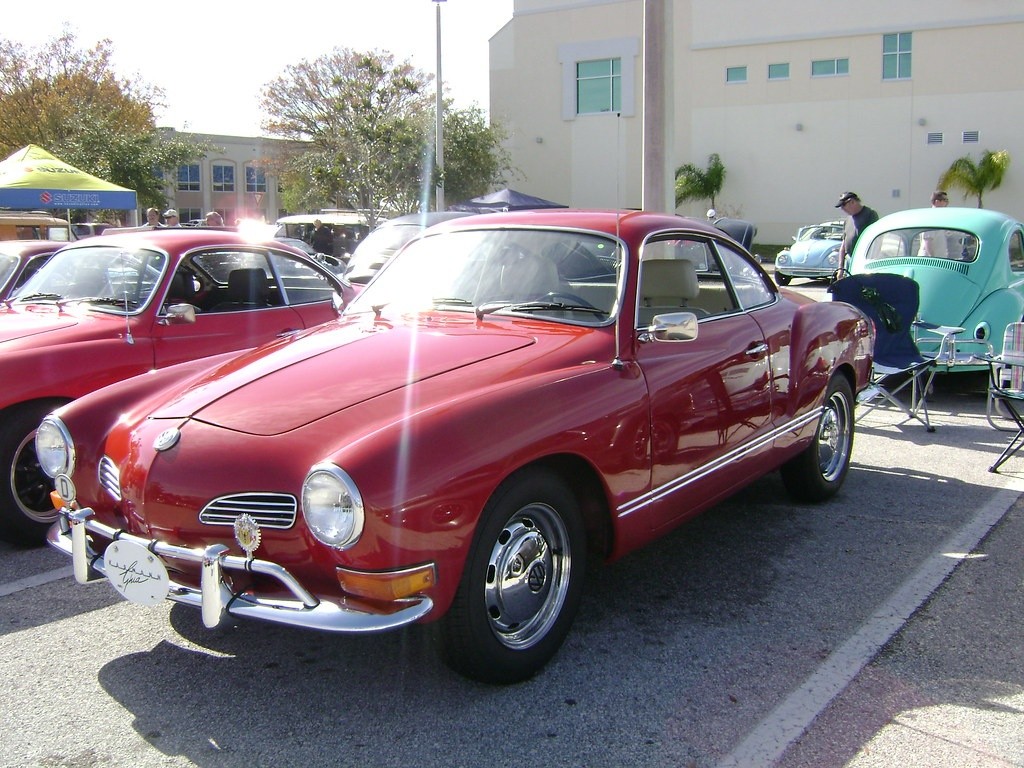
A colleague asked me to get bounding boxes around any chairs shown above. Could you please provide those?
[493,254,561,315]
[826,269,966,434]
[160,270,202,315]
[212,268,269,314]
[975,321,1024,474]
[636,259,711,330]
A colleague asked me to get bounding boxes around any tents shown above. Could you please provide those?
[451,188,569,212]
[0,144,140,241]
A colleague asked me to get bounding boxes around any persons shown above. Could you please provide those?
[706,209,718,220]
[835,192,883,281]
[310,219,334,256]
[163,209,182,227]
[141,208,165,227]
[918,191,950,259]
[206,212,226,226]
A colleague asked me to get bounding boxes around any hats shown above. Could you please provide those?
[162,209,179,217]
[834,191,857,207]
[707,209,716,217]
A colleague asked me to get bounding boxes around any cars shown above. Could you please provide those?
[1,229,367,551]
[253,237,347,277]
[35,209,880,688]
[0,239,200,302]
[774,221,845,287]
[846,208,1024,395]
[69,223,115,238]
[0,211,79,242]
[341,211,616,285]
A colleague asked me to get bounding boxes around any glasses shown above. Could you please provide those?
[843,198,850,207]
[165,215,176,219]
[938,198,948,202]
[709,215,715,219]
